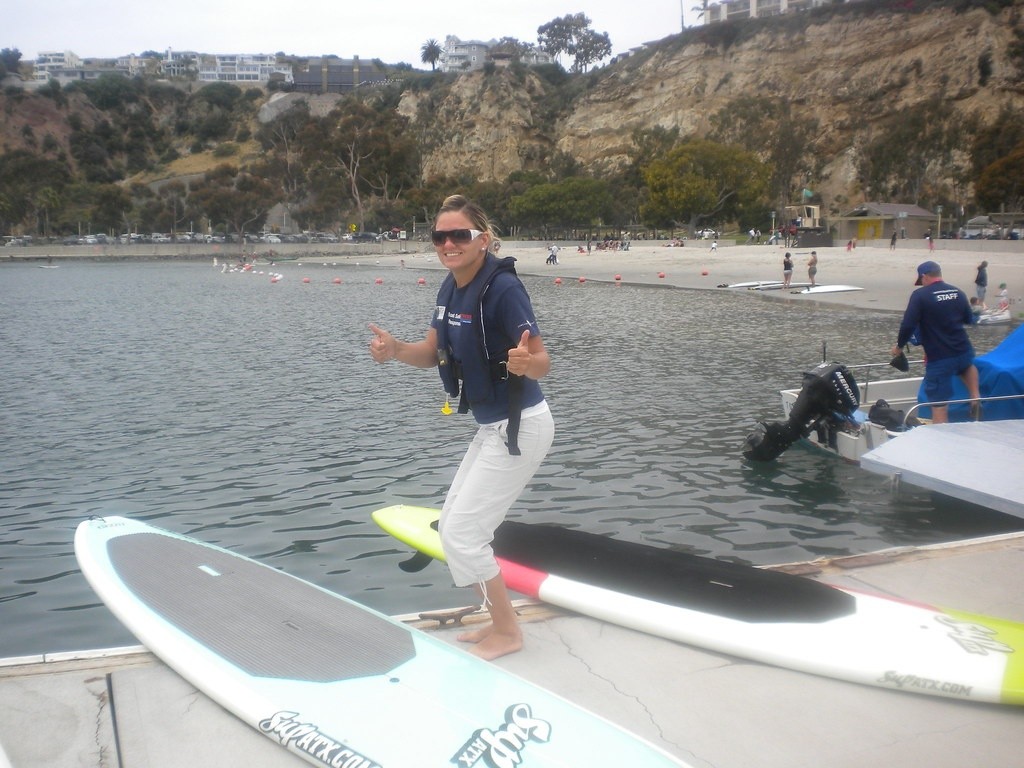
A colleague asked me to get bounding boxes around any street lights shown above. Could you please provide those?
[772,211,776,230]
[936,205,944,239]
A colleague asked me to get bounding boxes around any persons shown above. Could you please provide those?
[974,261,988,306]
[995,282,1008,310]
[781,252,794,290]
[890,232,897,250]
[552,243,558,264]
[927,224,1011,240]
[796,214,801,226]
[368,195,556,662]
[846,236,858,252]
[695,229,719,252]
[578,232,630,255]
[970,296,989,315]
[241,249,247,261]
[808,250,818,288]
[521,229,667,241]
[744,227,761,245]
[662,236,684,247]
[891,261,984,424]
[929,236,935,253]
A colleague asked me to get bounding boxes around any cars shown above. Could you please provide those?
[695,228,720,238]
[3,230,355,247]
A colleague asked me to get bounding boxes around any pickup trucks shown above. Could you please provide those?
[352,232,382,243]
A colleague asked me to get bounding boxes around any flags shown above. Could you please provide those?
[804,189,813,198]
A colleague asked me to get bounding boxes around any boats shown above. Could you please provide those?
[778,322,1024,462]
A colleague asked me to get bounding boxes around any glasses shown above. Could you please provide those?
[432,229,483,247]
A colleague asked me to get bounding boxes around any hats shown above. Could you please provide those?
[811,251,816,254]
[914,261,941,285]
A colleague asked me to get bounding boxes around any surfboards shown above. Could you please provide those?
[376,499,1022,709]
[751,283,821,290]
[73,513,697,768]
[799,285,864,294]
[728,281,785,288]
